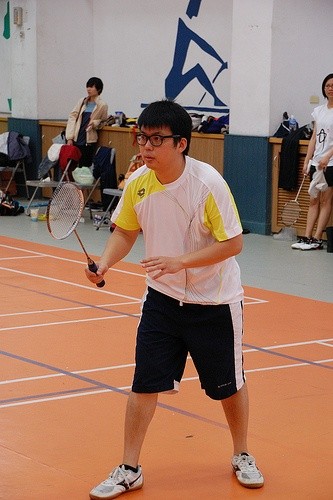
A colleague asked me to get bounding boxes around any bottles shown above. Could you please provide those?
[289,114,296,131]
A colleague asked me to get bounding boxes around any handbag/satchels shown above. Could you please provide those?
[118,161,146,191]
[0,191,24,216]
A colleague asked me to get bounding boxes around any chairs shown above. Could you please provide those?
[95,154,145,231]
[0,131,30,202]
[24,143,82,215]
[59,143,119,224]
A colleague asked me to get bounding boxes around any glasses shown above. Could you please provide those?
[135,132,180,146]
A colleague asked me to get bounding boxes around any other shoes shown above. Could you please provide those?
[85,199,103,211]
[93,214,110,226]
[274,228,297,241]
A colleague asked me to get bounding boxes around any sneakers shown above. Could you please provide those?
[231,452,264,488]
[89,465,143,500]
[291,236,324,250]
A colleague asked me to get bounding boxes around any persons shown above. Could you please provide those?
[66,77,108,169]
[291,74,333,251]
[84,101,264,500]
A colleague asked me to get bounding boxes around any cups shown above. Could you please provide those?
[31,208,39,222]
